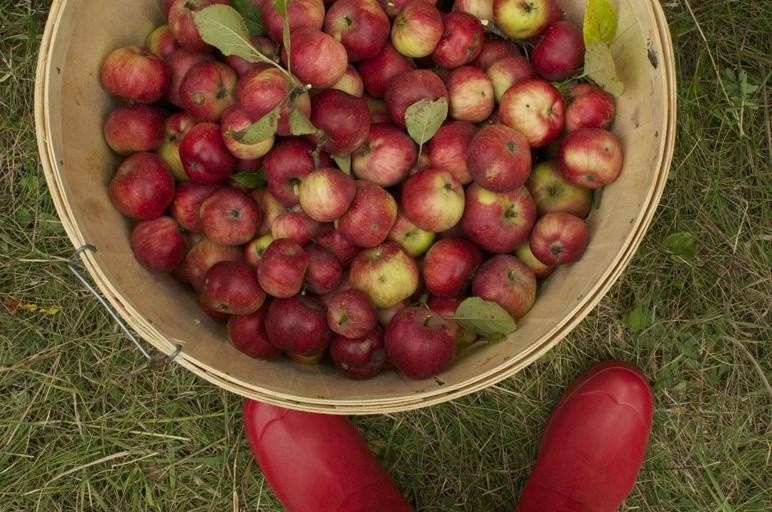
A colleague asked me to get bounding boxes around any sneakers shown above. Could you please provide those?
[513,360,657,511]
[238,396,421,512]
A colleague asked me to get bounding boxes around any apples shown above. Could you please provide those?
[101,0,623,379]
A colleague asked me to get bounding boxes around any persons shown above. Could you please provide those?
[242,357,655,511]
